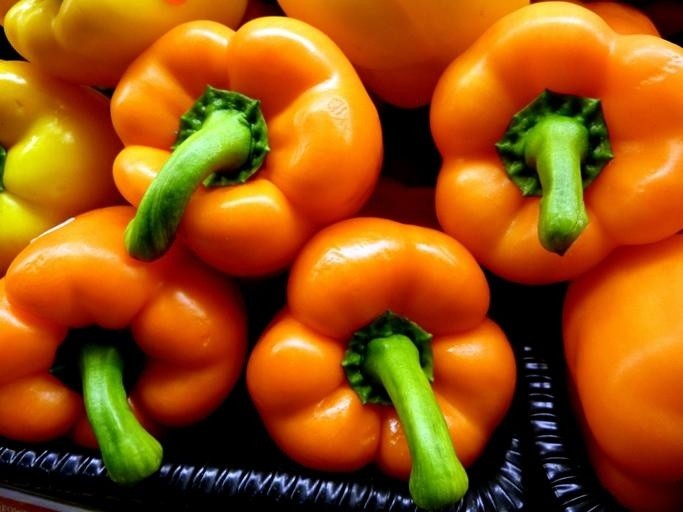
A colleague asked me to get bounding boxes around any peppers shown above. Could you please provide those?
[0,0,683,512]
